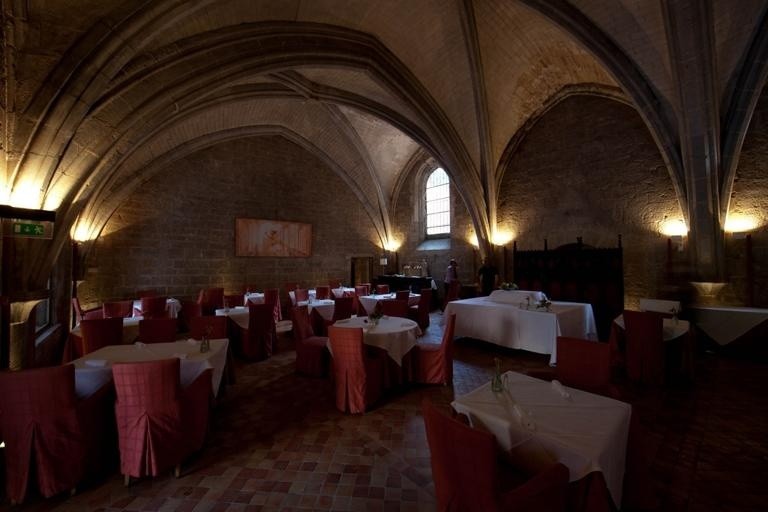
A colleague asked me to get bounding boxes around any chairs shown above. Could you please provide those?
[419,399,505,511]
[553,334,613,401]
[621,309,667,389]
[577,469,617,512]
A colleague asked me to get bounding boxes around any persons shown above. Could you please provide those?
[477,256,498,296]
[403,259,428,276]
[439,258,457,315]
[72,281,81,328]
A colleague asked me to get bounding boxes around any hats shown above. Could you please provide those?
[450,259,455,262]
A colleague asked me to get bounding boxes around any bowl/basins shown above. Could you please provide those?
[690,281,726,298]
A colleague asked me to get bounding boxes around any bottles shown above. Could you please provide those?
[492,372,503,390]
[504,373,509,390]
[520,295,552,312]
[199,336,210,352]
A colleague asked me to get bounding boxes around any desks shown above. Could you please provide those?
[607,312,689,397]
[445,369,634,511]
[443,289,599,368]
[690,305,768,364]
